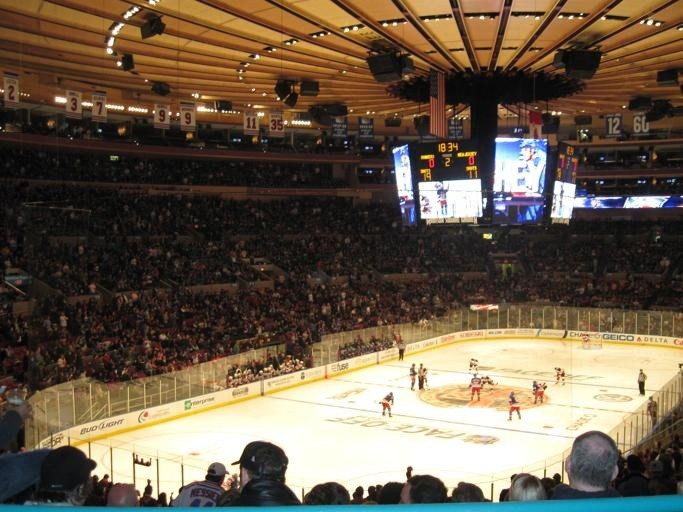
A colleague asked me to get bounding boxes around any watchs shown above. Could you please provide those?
[636,368,647,397]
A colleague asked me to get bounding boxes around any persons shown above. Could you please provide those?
[553,366,565,385]
[223,337,394,389]
[479,376,497,388]
[1,336,395,399]
[2,398,682,509]
[578,333,590,345]
[507,391,521,421]
[556,183,566,218]
[408,364,416,392]
[395,338,405,360]
[504,140,544,191]
[531,380,546,404]
[647,396,656,429]
[417,363,426,389]
[468,358,479,374]
[468,374,481,401]
[378,392,393,418]
[0,121,683,338]
[434,183,450,218]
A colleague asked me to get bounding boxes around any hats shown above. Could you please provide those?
[231,442,288,476]
[40,446,97,490]
[208,463,229,477]
